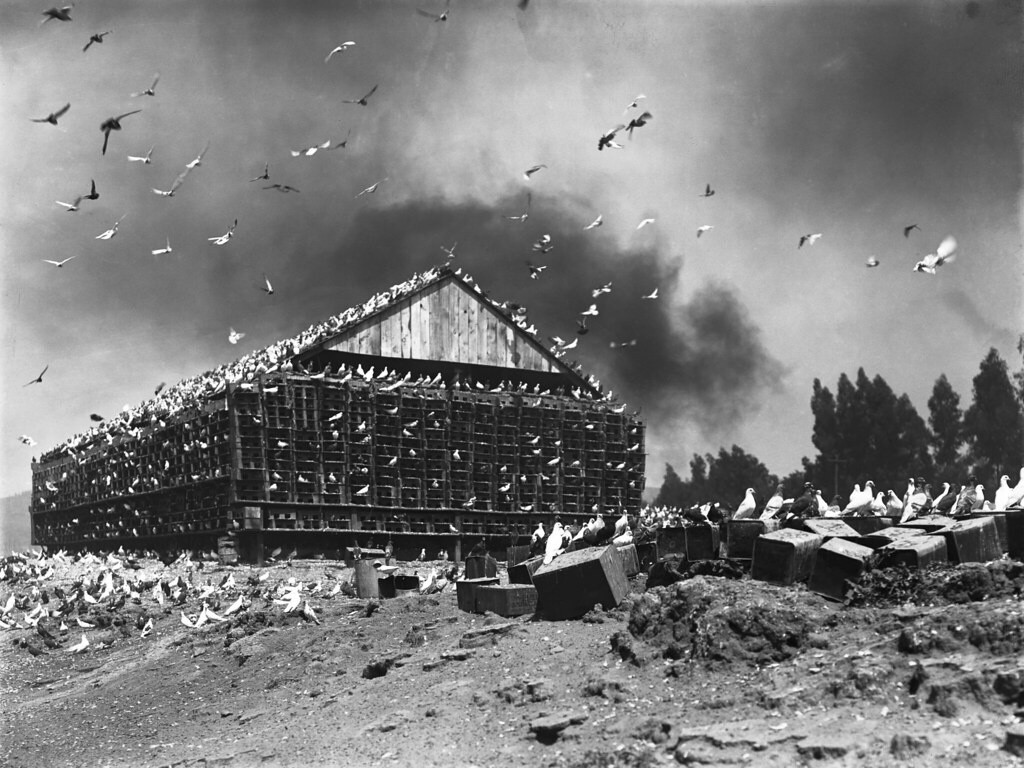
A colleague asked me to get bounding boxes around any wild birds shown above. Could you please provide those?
[28,102,71,126]
[129,73,159,97]
[41,162,388,295]
[341,84,378,106]
[0,261,1024,657]
[439,164,957,279]
[416,7,450,22]
[289,129,348,157]
[596,124,623,150]
[323,40,356,63]
[40,5,72,25]
[625,94,647,110]
[82,29,111,52]
[126,147,154,164]
[625,113,652,132]
[184,141,209,169]
[99,109,143,155]
